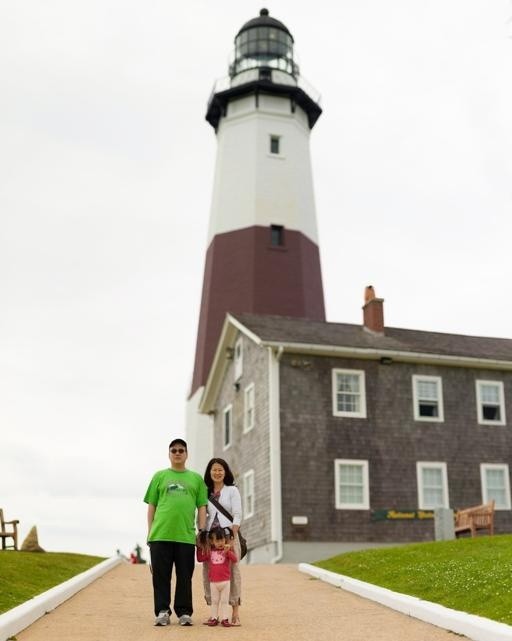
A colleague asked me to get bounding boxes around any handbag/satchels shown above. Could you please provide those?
[238,530,248,560]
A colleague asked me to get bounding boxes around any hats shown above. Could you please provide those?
[169,439,186,447]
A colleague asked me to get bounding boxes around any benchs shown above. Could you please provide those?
[453,499,496,538]
[0,508,19,550]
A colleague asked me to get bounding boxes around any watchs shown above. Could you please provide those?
[199,528,206,532]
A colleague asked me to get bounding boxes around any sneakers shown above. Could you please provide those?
[179,614,193,626]
[207,617,218,626]
[221,618,231,627]
[153,610,170,626]
[203,615,220,625]
[231,615,241,626]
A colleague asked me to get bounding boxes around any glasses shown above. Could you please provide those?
[170,448,184,454]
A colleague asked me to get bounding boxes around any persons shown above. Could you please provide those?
[197,527,238,627]
[196,458,242,626]
[144,439,212,626]
[134,543,142,559]
